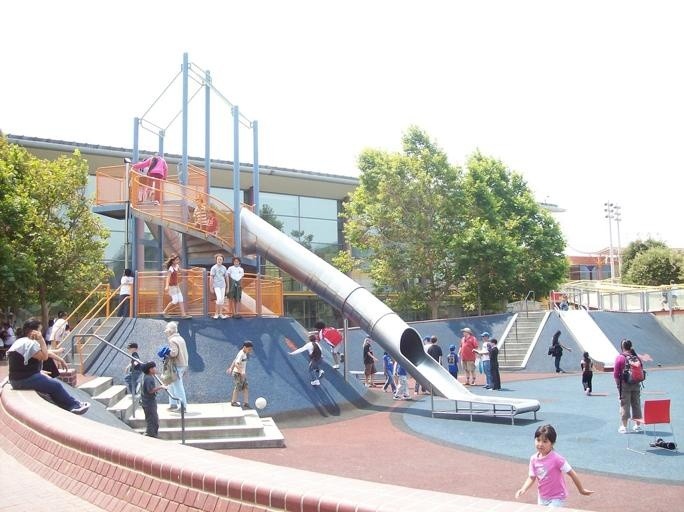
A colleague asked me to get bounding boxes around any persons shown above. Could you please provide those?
[613,338,642,434]
[193,198,219,239]
[288,334,325,386]
[209,254,229,319]
[661,280,680,311]
[0,312,91,414]
[551,330,572,373]
[226,340,253,410]
[315,320,345,369]
[559,291,570,311]
[124,321,188,437]
[227,256,245,318]
[580,352,594,396]
[118,267,134,317]
[130,152,169,206]
[160,251,192,320]
[515,424,594,508]
[363,327,502,401]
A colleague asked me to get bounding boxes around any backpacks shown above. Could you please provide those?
[621,354,647,385]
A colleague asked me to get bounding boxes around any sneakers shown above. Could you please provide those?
[617,425,629,435]
[339,353,345,363]
[585,387,590,394]
[632,424,643,433]
[70,401,91,415]
[332,364,340,369]
[162,311,172,319]
[318,369,324,378]
[213,313,220,319]
[167,405,178,412]
[231,401,241,407]
[242,402,252,410]
[403,395,412,401]
[181,314,193,320]
[392,395,402,401]
[174,407,187,413]
[310,379,321,386]
[219,313,227,320]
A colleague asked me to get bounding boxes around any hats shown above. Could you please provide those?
[480,332,490,336]
[460,328,473,334]
[448,344,456,351]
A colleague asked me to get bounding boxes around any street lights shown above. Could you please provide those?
[604,201,616,287]
[613,206,622,281]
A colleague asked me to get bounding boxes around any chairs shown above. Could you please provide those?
[626,399,680,457]
